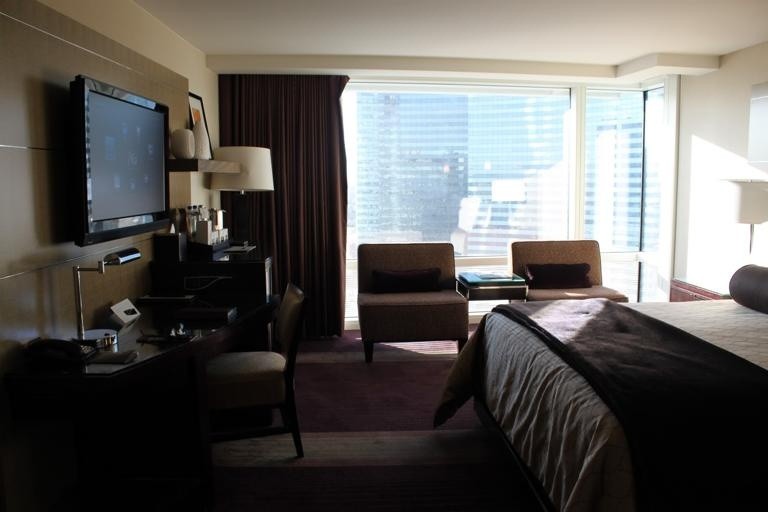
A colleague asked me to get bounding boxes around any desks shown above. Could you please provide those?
[77,295,281,511]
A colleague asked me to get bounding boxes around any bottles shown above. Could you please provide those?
[187,205,204,237]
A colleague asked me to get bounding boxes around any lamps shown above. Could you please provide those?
[71,247,143,349]
[728,178,768,255]
[208,145,275,253]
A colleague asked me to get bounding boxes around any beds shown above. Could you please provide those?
[471,300,767,510]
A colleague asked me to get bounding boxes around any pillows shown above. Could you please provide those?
[369,266,442,295]
[727,264,768,314]
[523,262,593,289]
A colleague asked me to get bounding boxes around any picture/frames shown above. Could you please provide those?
[188,92,214,159]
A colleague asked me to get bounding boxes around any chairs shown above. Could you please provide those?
[511,240,629,302]
[197,279,311,469]
[356,240,469,363]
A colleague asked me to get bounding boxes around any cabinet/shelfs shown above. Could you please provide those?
[147,253,273,345]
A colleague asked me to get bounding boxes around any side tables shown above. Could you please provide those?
[454,272,529,303]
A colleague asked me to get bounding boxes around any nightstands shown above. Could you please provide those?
[669,278,725,302]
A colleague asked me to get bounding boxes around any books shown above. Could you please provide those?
[472,269,512,279]
[458,271,525,287]
[223,245,257,253]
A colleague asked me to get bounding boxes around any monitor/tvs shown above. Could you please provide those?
[66,74,172,248]
